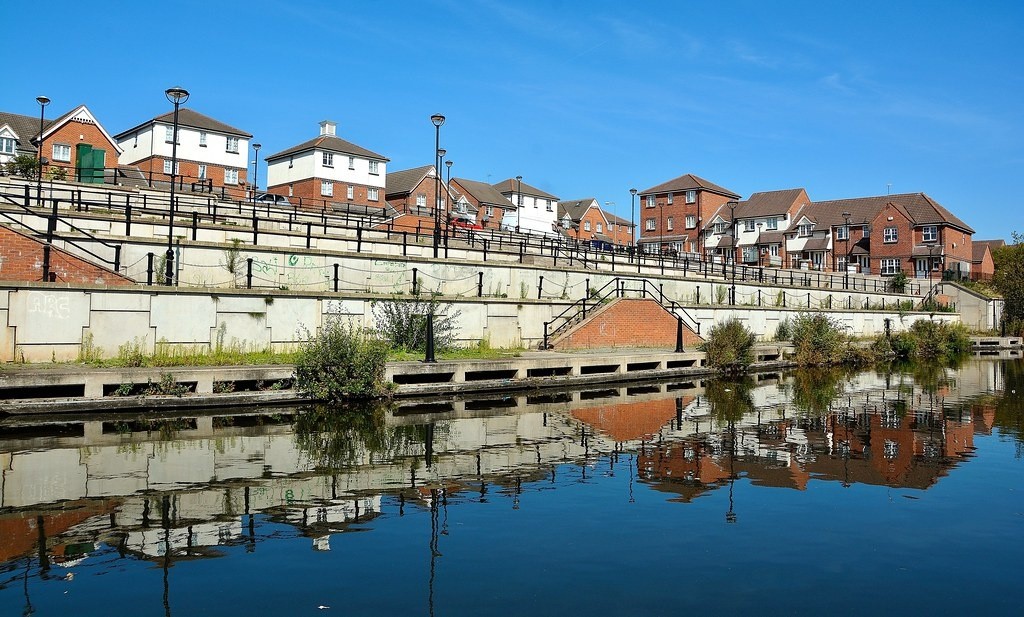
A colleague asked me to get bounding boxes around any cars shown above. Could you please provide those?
[244,192,293,207]
[447,216,485,229]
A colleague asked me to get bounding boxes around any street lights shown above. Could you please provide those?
[605,201,616,244]
[756,221,764,267]
[927,243,936,308]
[444,159,454,238]
[842,211,851,289]
[35,94,51,207]
[251,143,262,227]
[165,85,190,286]
[430,112,446,258]
[727,199,739,265]
[516,174,523,233]
[629,188,638,251]
[658,201,666,255]
[438,147,446,245]
